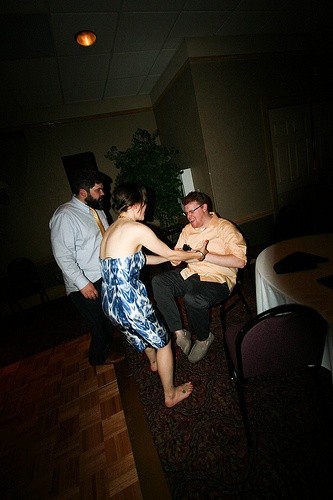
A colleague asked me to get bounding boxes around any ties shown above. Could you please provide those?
[90,206,105,237]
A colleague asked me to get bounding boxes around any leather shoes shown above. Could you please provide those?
[103,352,125,364]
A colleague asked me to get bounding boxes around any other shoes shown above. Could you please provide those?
[176,329,191,354]
[188,332,214,363]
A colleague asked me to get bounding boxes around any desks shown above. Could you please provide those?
[253,233,333,372]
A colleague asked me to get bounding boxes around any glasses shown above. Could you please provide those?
[182,203,204,216]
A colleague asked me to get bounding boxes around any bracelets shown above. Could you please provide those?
[196,250,205,261]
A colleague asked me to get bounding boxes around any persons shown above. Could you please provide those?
[100,182,209,407]
[49,170,125,364]
[152,192,247,364]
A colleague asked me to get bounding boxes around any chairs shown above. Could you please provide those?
[5,257,51,312]
[212,268,333,452]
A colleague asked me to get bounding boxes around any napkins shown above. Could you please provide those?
[273,249,329,275]
